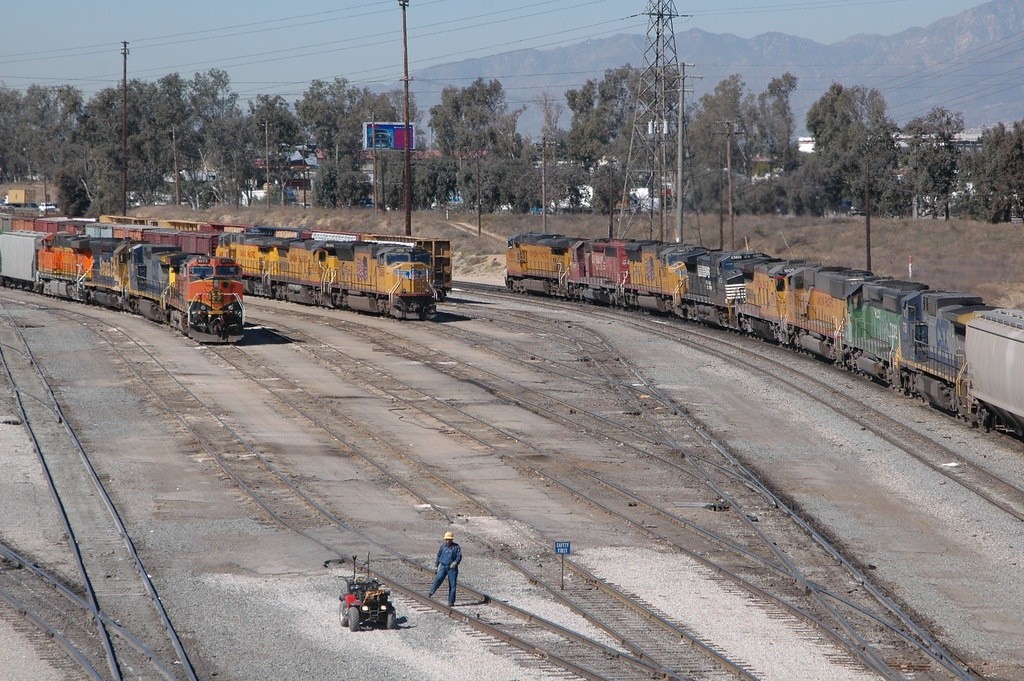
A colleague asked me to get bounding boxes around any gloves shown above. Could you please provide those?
[433,564,438,574]
[450,561,458,569]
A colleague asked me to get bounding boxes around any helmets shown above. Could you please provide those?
[444,531,454,539]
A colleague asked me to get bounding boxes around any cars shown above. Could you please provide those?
[39,202,56,214]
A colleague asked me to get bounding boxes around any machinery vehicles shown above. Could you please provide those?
[338,553,397,631]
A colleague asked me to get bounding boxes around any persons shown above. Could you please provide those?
[427,532,462,606]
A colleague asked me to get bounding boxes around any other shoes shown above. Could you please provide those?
[448,602,454,606]
[428,592,432,597]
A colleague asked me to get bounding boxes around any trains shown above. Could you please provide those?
[0,228,247,344]
[0,213,453,323]
[504,229,1024,440]
